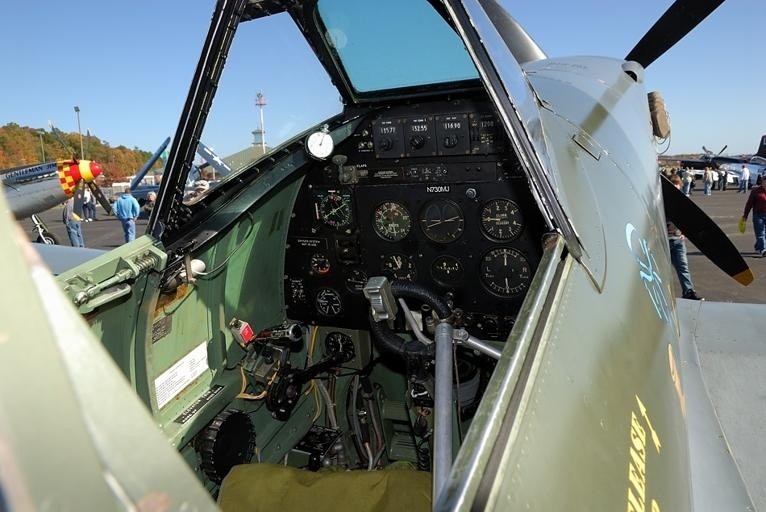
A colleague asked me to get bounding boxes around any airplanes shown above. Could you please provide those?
[2,158,104,221]
[0,0,764,512]
[680,144,766,186]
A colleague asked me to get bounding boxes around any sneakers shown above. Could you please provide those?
[681,290,702,300]
[761,249,766,257]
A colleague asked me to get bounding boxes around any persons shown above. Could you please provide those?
[182,180,210,201]
[112,188,141,243]
[62,187,100,223]
[200,166,208,180]
[663,175,705,301]
[142,191,157,217]
[63,197,85,248]
[742,176,766,256]
[659,165,730,197]
[737,165,750,193]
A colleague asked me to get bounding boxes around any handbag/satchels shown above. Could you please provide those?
[738,216,746,233]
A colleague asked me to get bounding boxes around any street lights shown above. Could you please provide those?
[72,105,85,158]
